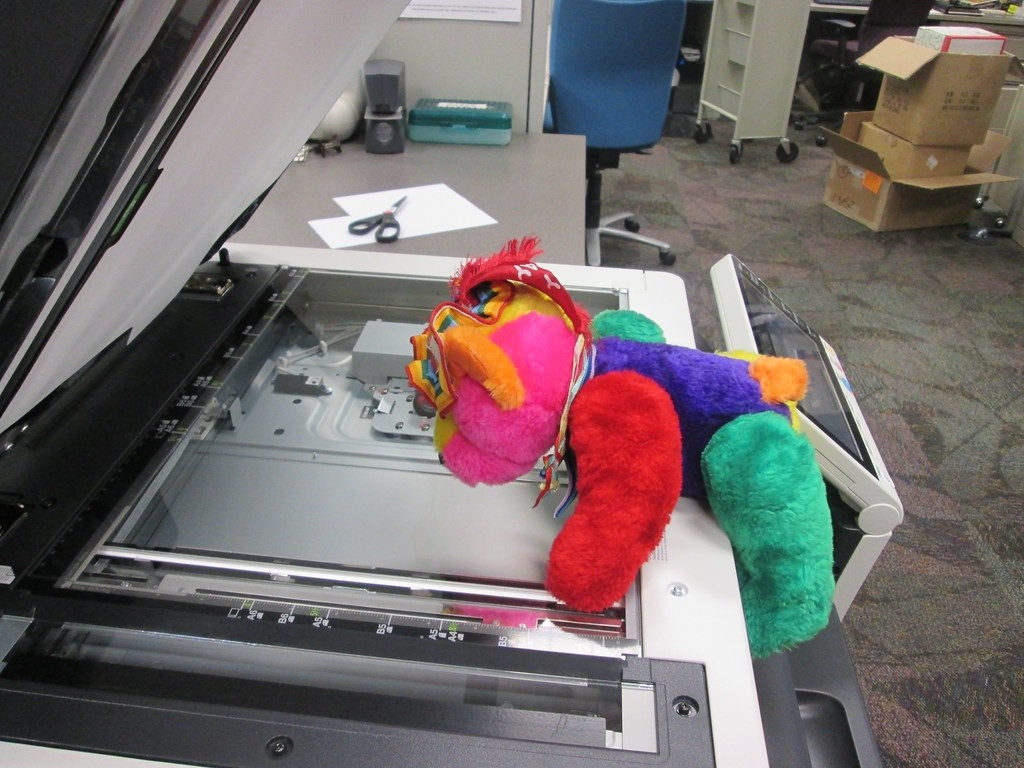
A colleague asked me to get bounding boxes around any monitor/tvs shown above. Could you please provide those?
[710,254,904,526]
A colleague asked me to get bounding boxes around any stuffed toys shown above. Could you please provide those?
[406,237,835,659]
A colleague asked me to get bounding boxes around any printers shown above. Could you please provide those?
[0,0,881,767]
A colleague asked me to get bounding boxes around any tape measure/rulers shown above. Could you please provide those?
[187,593,640,657]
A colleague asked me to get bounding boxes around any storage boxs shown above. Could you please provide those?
[855,36,1024,144]
[858,122,972,178]
[915,26,1007,54]
[819,112,1018,232]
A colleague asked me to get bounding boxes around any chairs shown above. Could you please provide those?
[542,0,686,267]
[794,0,936,147]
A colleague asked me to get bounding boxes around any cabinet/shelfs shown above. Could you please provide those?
[693,0,812,164]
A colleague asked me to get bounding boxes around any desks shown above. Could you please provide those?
[225,133,588,266]
[810,3,1024,26]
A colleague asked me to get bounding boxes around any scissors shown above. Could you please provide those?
[348,196,407,244]
